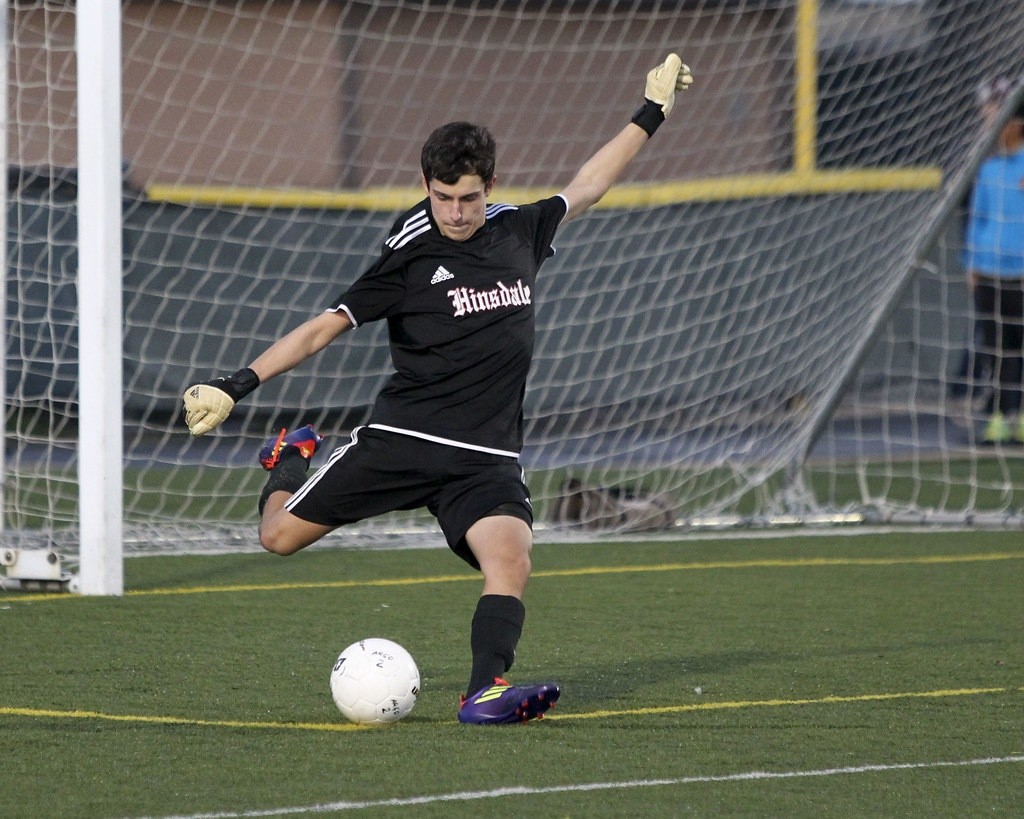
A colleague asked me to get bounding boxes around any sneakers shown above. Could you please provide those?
[257,424,324,470]
[457,677,561,726]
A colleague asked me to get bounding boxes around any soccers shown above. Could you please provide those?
[329,637,421,724]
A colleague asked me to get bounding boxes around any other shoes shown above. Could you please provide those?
[983,409,1024,444]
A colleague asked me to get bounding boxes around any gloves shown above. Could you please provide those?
[630,52,693,141]
[181,367,260,437]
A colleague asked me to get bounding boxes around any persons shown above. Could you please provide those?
[937,76,1024,446]
[183,52,694,726]
